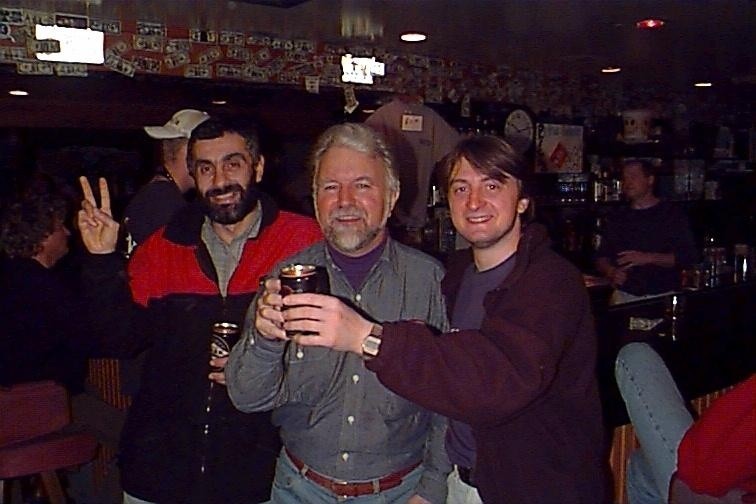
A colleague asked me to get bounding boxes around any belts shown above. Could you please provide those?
[284,447,422,497]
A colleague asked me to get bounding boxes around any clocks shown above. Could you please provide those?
[504,102,534,158]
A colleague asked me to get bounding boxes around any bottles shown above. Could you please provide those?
[589,215,607,258]
[587,154,625,202]
[732,243,750,284]
[454,113,497,136]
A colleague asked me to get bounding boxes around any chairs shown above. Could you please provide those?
[666,470,755,504]
[0,372,99,503]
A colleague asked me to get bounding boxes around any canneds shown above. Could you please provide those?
[280,263,320,340]
[208,321,241,373]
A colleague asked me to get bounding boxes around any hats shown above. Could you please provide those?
[144,110,209,139]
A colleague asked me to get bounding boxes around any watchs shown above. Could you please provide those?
[359,319,385,360]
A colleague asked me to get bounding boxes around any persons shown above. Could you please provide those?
[75,114,329,502]
[222,121,452,503]
[279,132,615,501]
[589,159,701,358]
[118,107,214,254]
[0,184,78,394]
